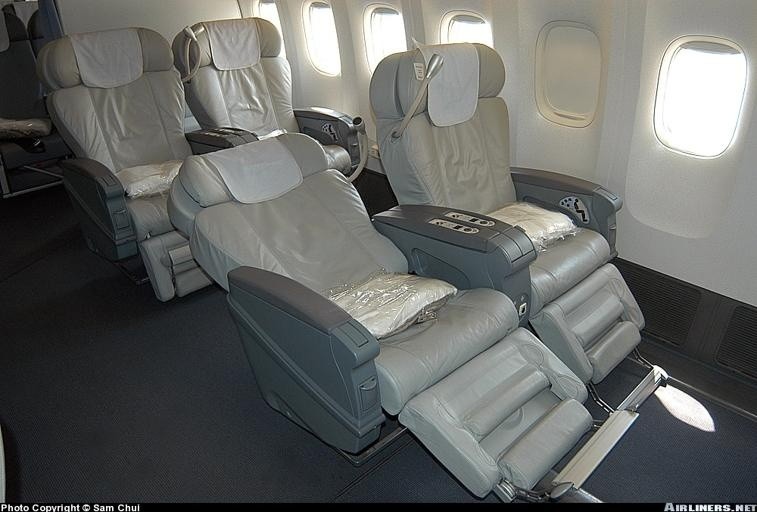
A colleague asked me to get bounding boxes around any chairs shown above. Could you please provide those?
[0,2,81,199]
[167,132,639,505]
[35,26,246,303]
[369,42,671,416]
[172,17,369,183]
